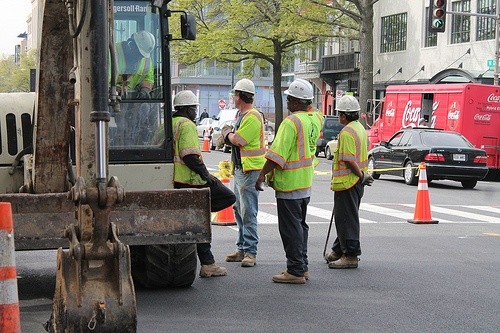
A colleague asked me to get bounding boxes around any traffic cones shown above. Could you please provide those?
[211,178,238,226]
[407,161,439,224]
[201,129,210,152]
[0,202,21,333]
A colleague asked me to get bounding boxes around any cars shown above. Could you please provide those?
[324,138,339,160]
[196,110,276,152]
[366,127,489,189]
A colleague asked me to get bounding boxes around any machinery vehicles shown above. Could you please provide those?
[0,0,211,333]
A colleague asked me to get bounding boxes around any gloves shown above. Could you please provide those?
[221,123,233,140]
[360,171,374,186]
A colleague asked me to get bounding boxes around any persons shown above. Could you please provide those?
[325,95,374,269]
[418,118,428,128]
[200,108,209,120]
[107,30,158,144]
[152,90,236,278]
[221,78,267,267]
[255,79,324,283]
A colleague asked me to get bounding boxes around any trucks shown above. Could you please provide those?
[360,82,500,181]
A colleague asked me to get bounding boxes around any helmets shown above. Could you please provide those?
[232,78,255,95]
[283,78,314,99]
[133,30,155,59]
[172,90,201,106]
[334,95,361,112]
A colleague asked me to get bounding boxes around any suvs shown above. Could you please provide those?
[314,114,346,157]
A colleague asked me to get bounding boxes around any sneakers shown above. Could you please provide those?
[241,253,256,267]
[225,250,245,262]
[199,263,227,277]
[325,251,341,263]
[328,254,360,268]
[272,271,309,284]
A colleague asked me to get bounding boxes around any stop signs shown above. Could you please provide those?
[218,99,226,109]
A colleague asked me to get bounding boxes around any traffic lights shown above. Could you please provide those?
[428,0,447,32]
